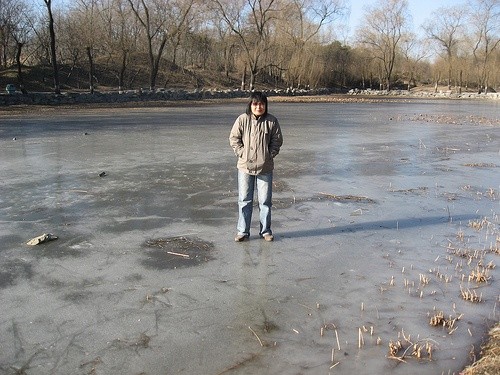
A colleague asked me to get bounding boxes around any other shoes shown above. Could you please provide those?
[262,232,273,242]
[235,235,249,242]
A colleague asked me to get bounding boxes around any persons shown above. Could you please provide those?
[229,92,283,242]
